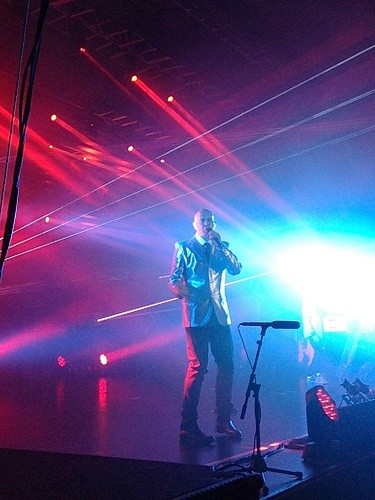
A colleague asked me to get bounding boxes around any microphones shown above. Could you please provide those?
[243,321,300,329]
[205,227,223,249]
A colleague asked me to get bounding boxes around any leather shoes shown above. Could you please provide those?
[216,415,243,437]
[180,416,216,443]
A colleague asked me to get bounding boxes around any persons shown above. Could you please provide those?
[167,208,244,443]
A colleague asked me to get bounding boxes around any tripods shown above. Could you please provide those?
[223,329,304,492]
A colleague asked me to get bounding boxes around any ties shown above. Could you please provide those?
[204,243,211,265]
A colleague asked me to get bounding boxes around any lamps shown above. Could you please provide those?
[340,377,374,409]
[302,386,348,461]
